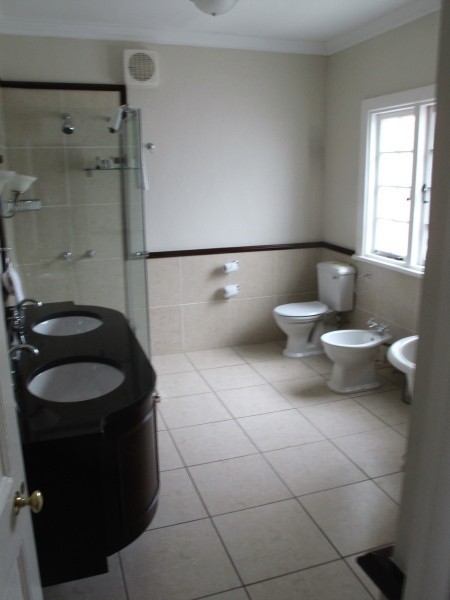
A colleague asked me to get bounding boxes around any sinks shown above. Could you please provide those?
[27,359,124,402]
[386,336,419,373]
[321,329,382,363]
[31,314,103,336]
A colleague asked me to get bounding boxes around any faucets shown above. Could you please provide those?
[367,318,384,335]
[7,345,38,360]
[9,299,42,339]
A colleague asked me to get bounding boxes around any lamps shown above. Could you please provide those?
[0,158,42,220]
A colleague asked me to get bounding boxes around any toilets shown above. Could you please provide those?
[272,261,356,358]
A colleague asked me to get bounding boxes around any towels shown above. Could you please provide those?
[2,268,23,302]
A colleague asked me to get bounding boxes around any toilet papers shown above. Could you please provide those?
[222,284,240,298]
[222,261,240,274]
